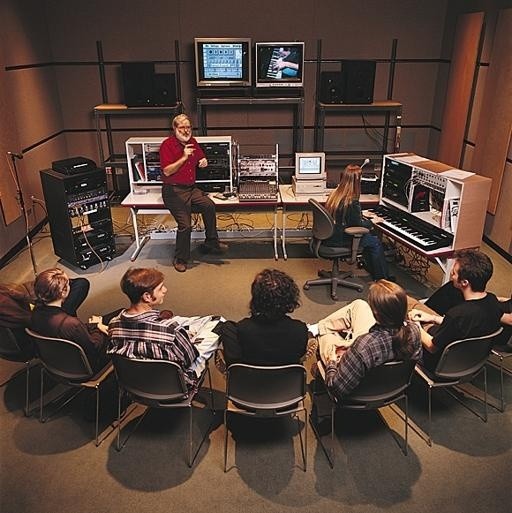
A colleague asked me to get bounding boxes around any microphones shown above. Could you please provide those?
[359,158,369,170]
[7,151,24,160]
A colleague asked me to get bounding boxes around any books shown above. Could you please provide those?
[162,314,227,354]
[131,155,146,182]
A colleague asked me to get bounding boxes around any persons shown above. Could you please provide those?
[272,48,300,77]
[322,163,397,281]
[31,268,126,383]
[222,269,308,414]
[107,268,213,403]
[0,278,90,361]
[159,113,229,272]
[306,278,421,406]
[407,249,504,384]
[424,280,512,346]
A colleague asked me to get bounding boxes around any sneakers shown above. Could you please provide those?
[299,338,318,365]
[213,350,226,375]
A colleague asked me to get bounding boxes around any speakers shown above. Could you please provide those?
[155,74,176,106]
[340,60,377,102]
[118,62,153,106]
[321,72,339,104]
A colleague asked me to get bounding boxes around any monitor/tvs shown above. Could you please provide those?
[254,41,305,88]
[193,36,252,88]
[295,152,324,180]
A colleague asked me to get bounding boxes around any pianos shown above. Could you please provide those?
[362,204,454,251]
[267,48,284,78]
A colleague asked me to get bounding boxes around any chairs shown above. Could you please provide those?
[221,363,309,474]
[413,326,505,448]
[24,327,138,448]
[109,353,218,468]
[0,325,77,418]
[309,358,417,471]
[490,347,512,414]
[302,198,364,302]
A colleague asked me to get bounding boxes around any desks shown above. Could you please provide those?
[119,191,282,263]
[277,183,380,262]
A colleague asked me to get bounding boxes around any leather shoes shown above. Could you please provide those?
[205,242,228,249]
[173,257,187,271]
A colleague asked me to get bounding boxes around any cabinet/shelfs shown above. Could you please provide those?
[92,102,185,207]
[315,101,405,168]
[361,151,493,305]
[195,97,306,171]
